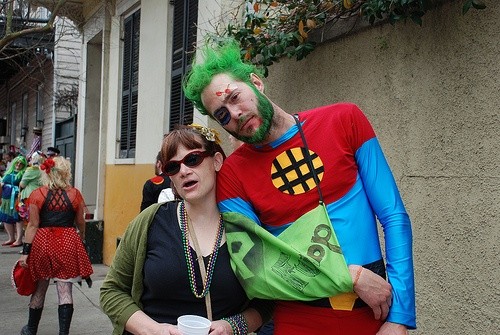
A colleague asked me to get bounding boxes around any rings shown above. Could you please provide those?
[21,261,24,264]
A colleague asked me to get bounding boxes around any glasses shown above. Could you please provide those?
[162,150,209,176]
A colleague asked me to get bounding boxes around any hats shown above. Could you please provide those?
[32,127,42,133]
[46,147,60,155]
[11,259,36,296]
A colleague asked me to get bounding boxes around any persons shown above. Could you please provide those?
[140,152,176,212]
[15,150,58,224]
[0,155,27,247]
[11,155,94,335]
[0,147,61,178]
[181,38,417,335]
[101,123,277,335]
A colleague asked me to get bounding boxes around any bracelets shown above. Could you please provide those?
[222,314,248,335]
[354,266,363,287]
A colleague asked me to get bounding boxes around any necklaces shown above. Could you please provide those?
[180,200,224,298]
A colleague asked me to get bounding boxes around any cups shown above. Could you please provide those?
[177,315,212,335]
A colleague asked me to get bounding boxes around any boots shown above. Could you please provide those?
[21,307,43,335]
[58,303,74,335]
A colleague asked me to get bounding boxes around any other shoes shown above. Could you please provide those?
[2,240,16,246]
[10,241,23,247]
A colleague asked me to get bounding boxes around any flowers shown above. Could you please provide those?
[39,158,56,174]
